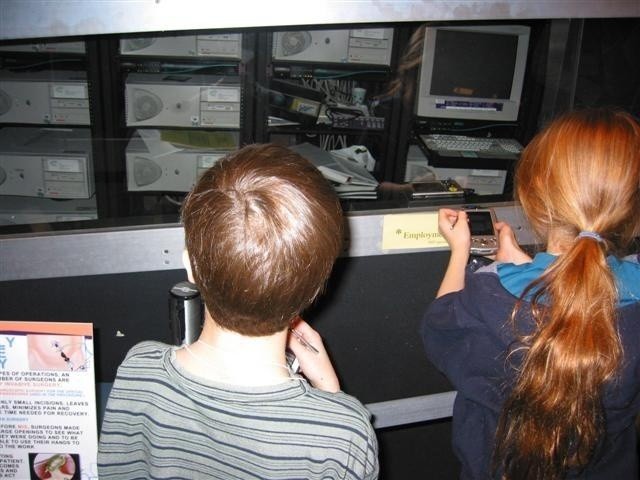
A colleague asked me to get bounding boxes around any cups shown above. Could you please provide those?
[169,283,200,346]
[351,87,366,104]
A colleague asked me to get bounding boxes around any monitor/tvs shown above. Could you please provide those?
[413,26,532,122]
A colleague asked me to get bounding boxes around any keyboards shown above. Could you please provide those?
[418,131,524,161]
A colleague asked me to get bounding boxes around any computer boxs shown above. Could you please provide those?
[118,34,242,60]
[0,68,91,126]
[403,144,508,198]
[270,28,395,68]
[0,127,96,201]
[122,72,239,131]
[124,128,239,193]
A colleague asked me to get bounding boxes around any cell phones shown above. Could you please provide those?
[462,206,500,257]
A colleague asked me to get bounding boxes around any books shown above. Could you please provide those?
[289,141,378,201]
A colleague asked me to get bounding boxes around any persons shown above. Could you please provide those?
[420,107,640,480]
[97,144,379,480]
[369,28,456,197]
